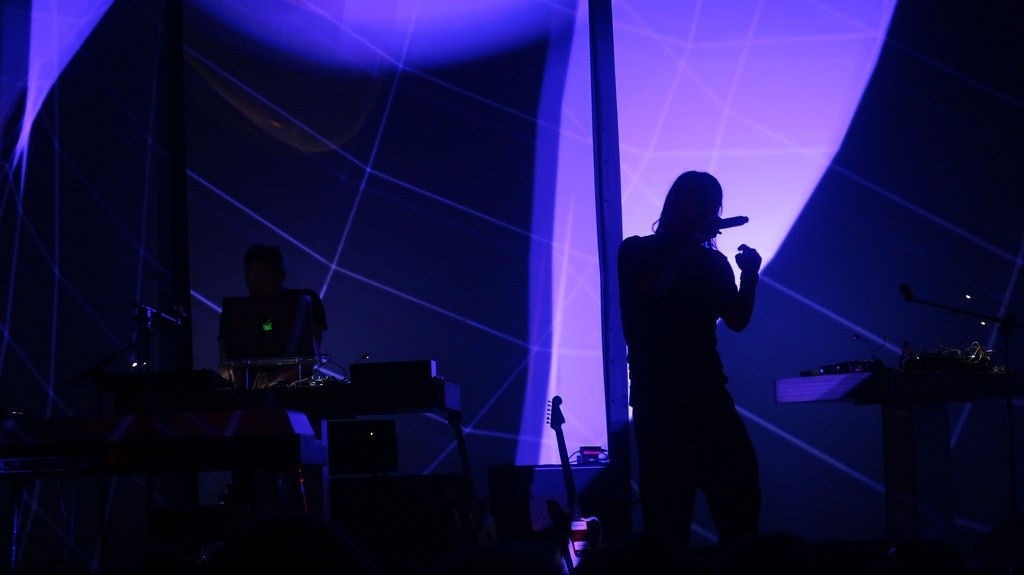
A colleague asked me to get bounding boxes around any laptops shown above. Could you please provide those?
[222,293,320,360]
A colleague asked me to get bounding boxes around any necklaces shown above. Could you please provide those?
[263,319,273,330]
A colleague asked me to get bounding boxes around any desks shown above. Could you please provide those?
[774,373,1023,544]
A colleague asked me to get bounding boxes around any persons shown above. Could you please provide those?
[218,245,326,390]
[617,171,761,547]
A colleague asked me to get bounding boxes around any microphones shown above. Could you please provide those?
[719,215,749,230]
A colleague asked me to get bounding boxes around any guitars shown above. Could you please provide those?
[544,393,600,574]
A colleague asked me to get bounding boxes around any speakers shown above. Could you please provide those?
[488,463,606,539]
[328,419,479,559]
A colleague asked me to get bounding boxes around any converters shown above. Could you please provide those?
[576,446,601,464]
